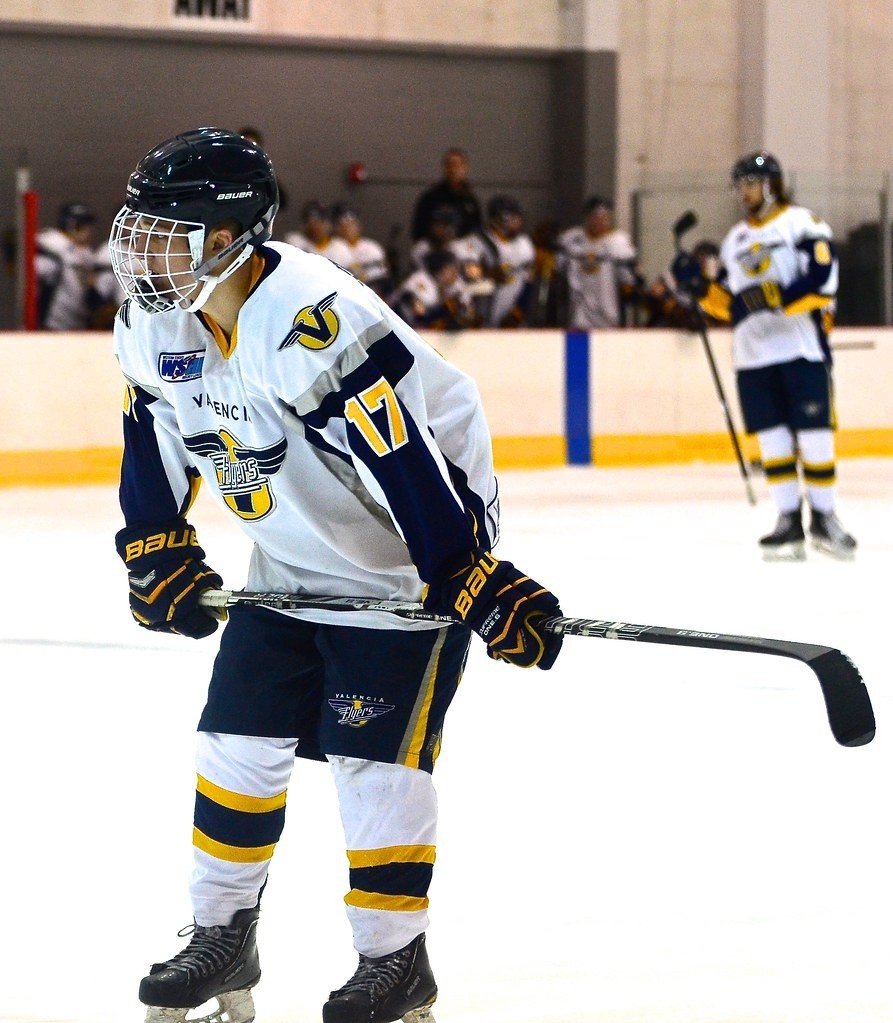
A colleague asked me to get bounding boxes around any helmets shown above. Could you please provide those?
[106,126,280,316]
[731,151,781,186]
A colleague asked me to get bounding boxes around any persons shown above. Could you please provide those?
[670,153,860,561]
[116,128,566,1023]
[1,145,732,332]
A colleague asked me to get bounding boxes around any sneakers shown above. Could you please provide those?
[757,497,806,562]
[809,508,858,561]
[322,932,438,1023]
[139,873,269,1023]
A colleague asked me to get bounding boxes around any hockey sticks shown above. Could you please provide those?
[191,583,878,746]
[669,206,760,509]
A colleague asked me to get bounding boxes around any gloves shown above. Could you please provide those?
[114,518,228,640]
[671,252,710,297]
[421,547,564,670]
